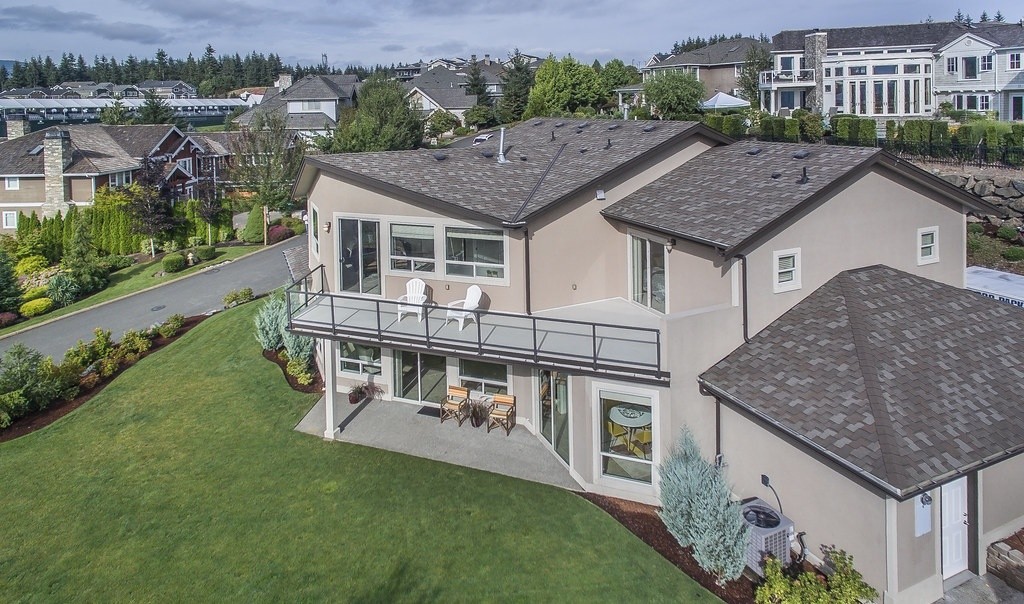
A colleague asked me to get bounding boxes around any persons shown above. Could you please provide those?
[302,212,308,232]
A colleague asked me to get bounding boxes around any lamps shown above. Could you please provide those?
[920,494,933,506]
[323,221,330,233]
[665,238,676,254]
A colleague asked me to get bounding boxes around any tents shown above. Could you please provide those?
[698,90,751,116]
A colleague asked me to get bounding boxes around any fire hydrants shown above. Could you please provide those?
[187,254,194,265]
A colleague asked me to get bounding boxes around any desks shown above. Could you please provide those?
[608,404,652,451]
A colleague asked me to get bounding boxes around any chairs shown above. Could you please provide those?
[607,419,627,447]
[440,385,469,427]
[357,346,381,373]
[630,430,652,459]
[445,285,482,332]
[397,277,427,323]
[486,394,515,436]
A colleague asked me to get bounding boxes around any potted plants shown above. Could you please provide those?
[349,383,385,404]
[460,399,491,429]
